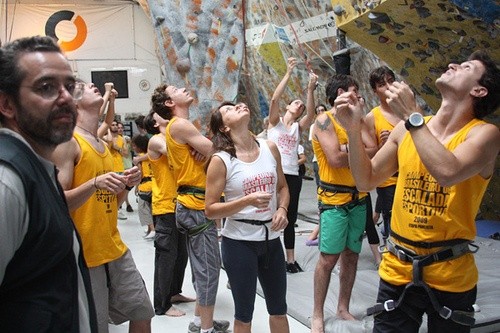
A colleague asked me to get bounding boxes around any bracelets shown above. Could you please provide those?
[125,185,134,191]
[280,206,288,212]
[95,178,99,189]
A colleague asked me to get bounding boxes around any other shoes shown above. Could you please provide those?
[127,205,133,212]
[117,209,127,219]
[145,230,155,239]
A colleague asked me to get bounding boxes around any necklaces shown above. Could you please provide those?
[76,125,100,142]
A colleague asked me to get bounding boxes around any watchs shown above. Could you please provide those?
[118,148,121,152]
[405,112,424,129]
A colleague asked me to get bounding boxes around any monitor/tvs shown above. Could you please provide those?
[90,71,129,99]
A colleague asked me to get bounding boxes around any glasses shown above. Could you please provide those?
[16,83,85,101]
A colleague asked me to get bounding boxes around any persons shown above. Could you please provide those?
[145,110,197,316]
[365,65,425,244]
[205,120,233,289]
[309,104,328,186]
[258,57,317,273]
[51,78,156,333]
[334,49,500,333]
[311,74,378,333]
[0,35,100,333]
[152,85,233,333]
[204,102,290,333]
[98,82,155,239]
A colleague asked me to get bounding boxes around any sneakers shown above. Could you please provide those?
[187,320,230,333]
[285,260,303,273]
